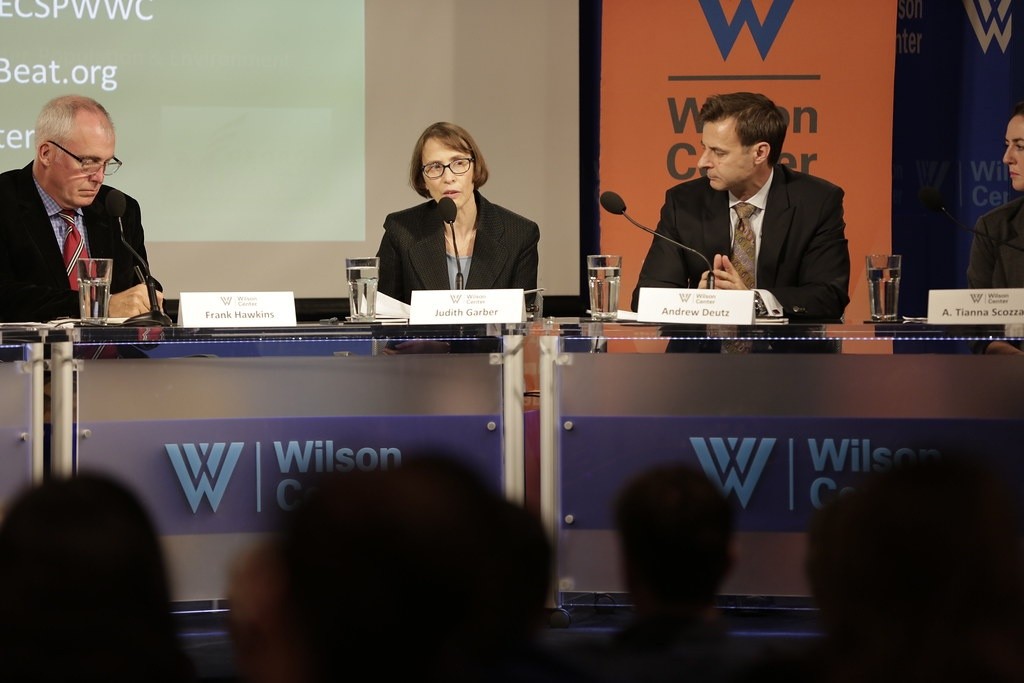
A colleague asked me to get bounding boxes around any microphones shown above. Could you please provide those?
[105,190,172,328]
[437,196,464,290]
[598,191,715,289]
[918,187,1024,251]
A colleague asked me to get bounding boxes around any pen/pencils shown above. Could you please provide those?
[134,266,146,285]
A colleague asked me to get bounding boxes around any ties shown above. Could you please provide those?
[705,324,737,338]
[135,325,163,341]
[720,202,756,352]
[57,209,120,359]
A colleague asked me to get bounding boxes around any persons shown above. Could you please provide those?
[967,101,1024,289]
[377,122,540,308]
[229,454,555,683]
[567,461,743,683]
[632,92,851,324]
[0,473,194,683]
[807,449,1024,683]
[0,95,164,323]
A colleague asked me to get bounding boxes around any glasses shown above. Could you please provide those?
[45,140,122,176]
[424,158,474,179]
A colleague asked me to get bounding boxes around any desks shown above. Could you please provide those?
[530,312,1024,627]
[46,317,528,627]
[0,319,46,520]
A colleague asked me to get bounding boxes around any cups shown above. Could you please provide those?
[865,254,902,321]
[76,257,113,325]
[345,257,379,321]
[587,254,621,320]
